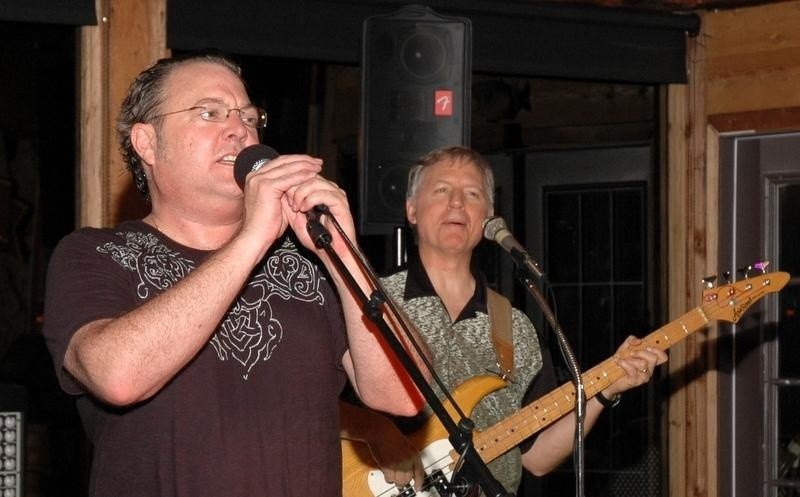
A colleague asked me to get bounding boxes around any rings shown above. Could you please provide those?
[640,369,647,374]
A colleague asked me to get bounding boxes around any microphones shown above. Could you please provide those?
[234,143,336,249]
[481,215,546,281]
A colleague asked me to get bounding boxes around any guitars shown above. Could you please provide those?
[341,261,790,497]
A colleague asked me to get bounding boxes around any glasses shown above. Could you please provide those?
[159,106,268,128]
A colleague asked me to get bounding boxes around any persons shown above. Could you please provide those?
[41,54,432,497]
[341,147,669,497]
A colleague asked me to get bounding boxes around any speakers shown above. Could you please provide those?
[358,6,473,234]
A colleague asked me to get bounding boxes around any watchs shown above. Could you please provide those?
[595,391,621,408]
